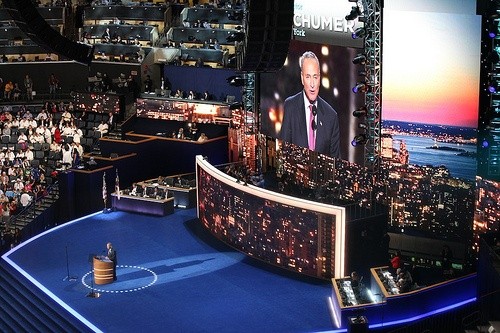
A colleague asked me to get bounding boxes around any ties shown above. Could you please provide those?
[309,105,316,151]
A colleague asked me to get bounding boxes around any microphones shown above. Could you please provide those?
[103,251,105,252]
[312,105,317,116]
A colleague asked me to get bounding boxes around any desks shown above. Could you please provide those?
[91,61,142,96]
[67,165,114,218]
[136,182,196,209]
[181,49,224,62]
[84,25,153,41]
[164,64,242,102]
[188,7,243,24]
[126,131,227,175]
[172,27,245,45]
[332,276,362,328]
[0,62,90,96]
[83,152,136,191]
[110,191,174,216]
[136,96,241,124]
[76,91,125,115]
[100,136,158,182]
[370,267,403,322]
[0,6,70,54]
[94,44,152,57]
[93,6,166,21]
[479,234,500,276]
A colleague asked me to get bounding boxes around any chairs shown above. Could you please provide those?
[0,106,110,189]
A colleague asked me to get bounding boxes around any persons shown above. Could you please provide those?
[351,272,358,286]
[280,51,341,160]
[158,175,184,188]
[0,101,115,232]
[173,128,208,142]
[390,251,408,293]
[107,242,117,281]
[0,0,244,103]
[129,182,172,199]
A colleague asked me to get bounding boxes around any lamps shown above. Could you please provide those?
[226,76,249,87]
[228,102,244,110]
[345,7,372,147]
[483,25,497,147]
